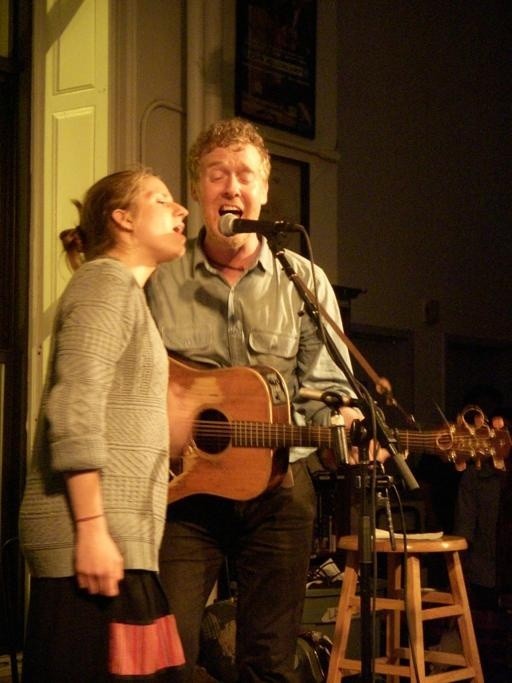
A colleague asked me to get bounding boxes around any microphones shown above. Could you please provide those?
[217,212,297,237]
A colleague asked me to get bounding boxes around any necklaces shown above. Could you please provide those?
[204,256,244,273]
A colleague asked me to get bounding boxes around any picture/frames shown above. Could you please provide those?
[232,1,317,257]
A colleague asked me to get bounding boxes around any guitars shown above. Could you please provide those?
[169,350,510,505]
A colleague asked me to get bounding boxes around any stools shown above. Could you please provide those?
[326,533,483,683]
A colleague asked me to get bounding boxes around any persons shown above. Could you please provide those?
[449,454,512,642]
[144,118,390,682]
[15,170,192,683]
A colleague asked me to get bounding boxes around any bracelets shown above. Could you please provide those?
[75,511,105,526]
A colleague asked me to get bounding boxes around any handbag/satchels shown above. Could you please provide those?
[306,558,360,587]
